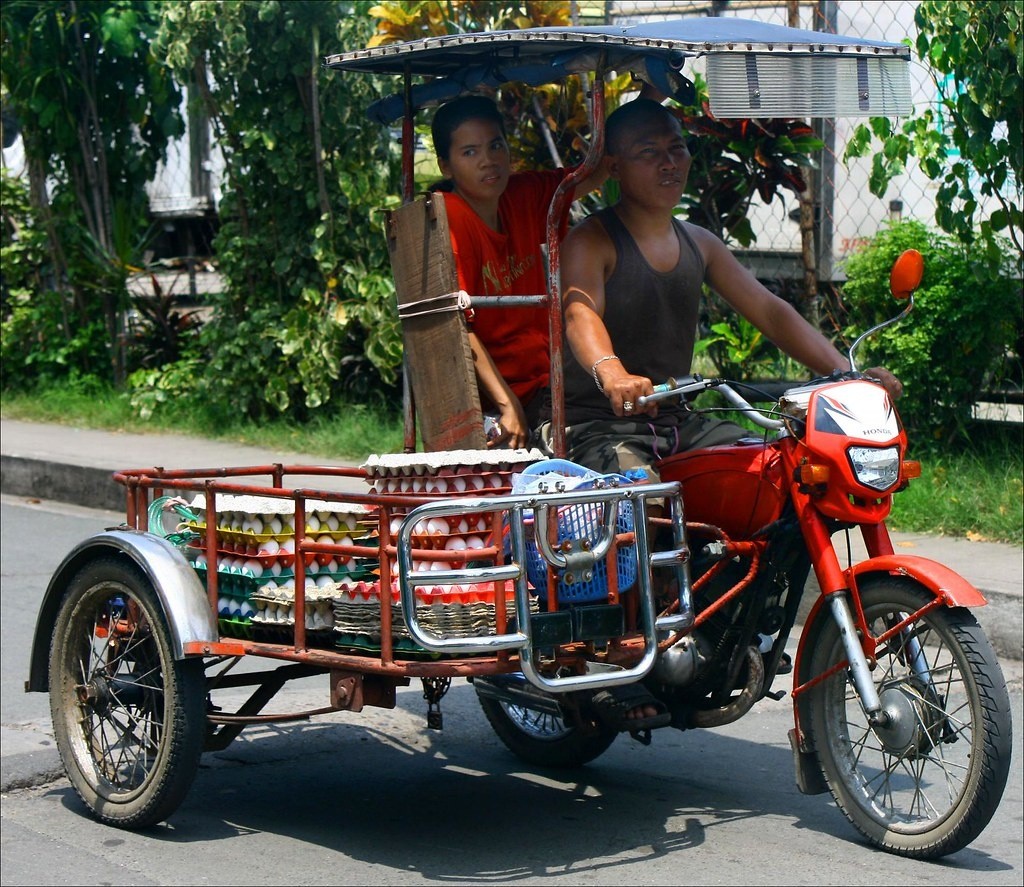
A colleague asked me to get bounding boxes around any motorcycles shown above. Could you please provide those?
[22,13,1015,859]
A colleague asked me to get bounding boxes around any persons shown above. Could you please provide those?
[431,84,675,451]
[560,97,902,730]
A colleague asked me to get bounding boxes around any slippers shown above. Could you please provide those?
[579,684,672,731]
[762,651,793,673]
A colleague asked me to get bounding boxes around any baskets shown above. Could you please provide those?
[508,459,637,602]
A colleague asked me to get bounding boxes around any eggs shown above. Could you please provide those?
[195,473,525,625]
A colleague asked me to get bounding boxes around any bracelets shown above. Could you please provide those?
[592,355,620,392]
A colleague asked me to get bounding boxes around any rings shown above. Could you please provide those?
[624,401,636,412]
